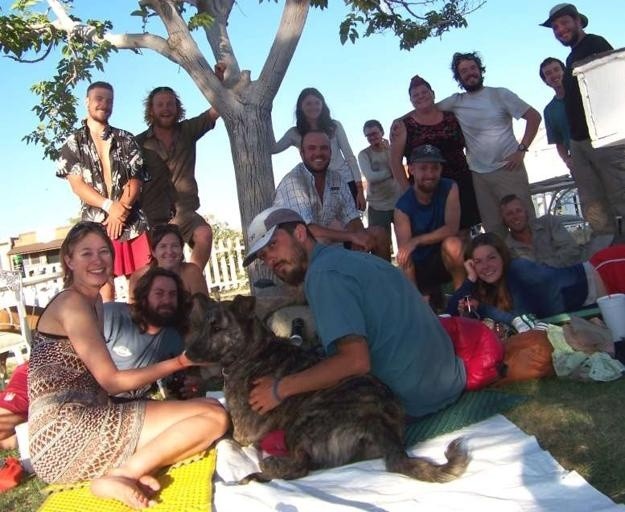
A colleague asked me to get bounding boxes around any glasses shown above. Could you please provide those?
[151,223,178,232]
[464,294,472,312]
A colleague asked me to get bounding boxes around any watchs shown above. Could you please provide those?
[518,144,526,151]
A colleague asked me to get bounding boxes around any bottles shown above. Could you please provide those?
[463,295,475,319]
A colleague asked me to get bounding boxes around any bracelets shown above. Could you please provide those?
[274,378,283,401]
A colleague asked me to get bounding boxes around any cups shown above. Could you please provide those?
[596,293,625,344]
[14,421,30,460]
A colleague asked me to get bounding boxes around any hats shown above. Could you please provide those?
[242,207,305,266]
[410,144,446,163]
[539,3,588,30]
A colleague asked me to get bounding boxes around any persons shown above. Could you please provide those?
[242,205,466,420]
[25,220,231,507]
[136,61,227,271]
[129,223,209,311]
[358,120,405,261]
[393,144,473,307]
[539,57,573,173]
[274,129,377,254]
[0,267,186,452]
[390,52,541,240]
[541,3,625,239]
[446,231,625,323]
[272,88,367,212]
[389,75,482,233]
[498,194,582,269]
[56,81,152,303]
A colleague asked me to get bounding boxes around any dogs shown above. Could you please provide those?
[183,293,475,485]
